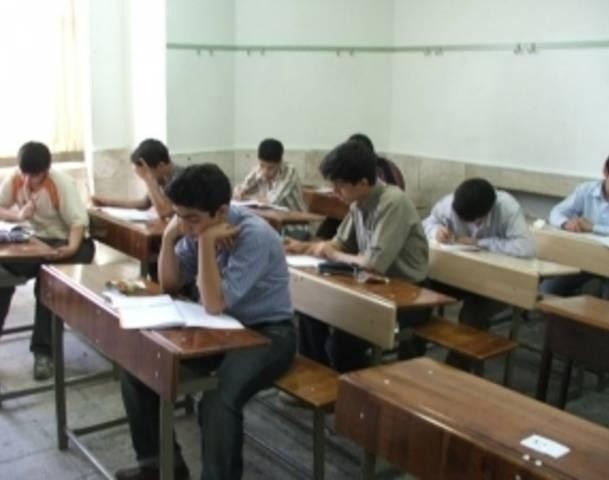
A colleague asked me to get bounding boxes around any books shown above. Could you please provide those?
[235,198,283,210]
[115,294,245,331]
[103,203,160,221]
[1,221,28,240]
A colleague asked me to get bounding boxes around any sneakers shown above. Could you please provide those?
[112,456,191,480]
[33,354,54,381]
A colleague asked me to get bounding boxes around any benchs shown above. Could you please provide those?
[276,353,345,479]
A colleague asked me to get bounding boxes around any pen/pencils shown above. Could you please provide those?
[282,229,286,244]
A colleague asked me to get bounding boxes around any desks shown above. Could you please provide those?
[248,189,609,404]
[336,356,609,480]
[36,232,271,479]
[90,208,160,269]
[0,236,53,336]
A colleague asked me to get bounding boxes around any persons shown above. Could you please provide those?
[112,158,305,480]
[395,177,535,370]
[540,154,609,308]
[0,140,97,382]
[232,135,314,240]
[86,135,188,225]
[281,137,430,369]
[347,131,408,195]
